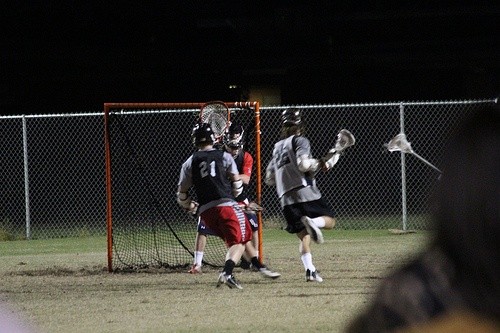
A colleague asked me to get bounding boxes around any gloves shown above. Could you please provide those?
[186,201,200,215]
[319,148,341,172]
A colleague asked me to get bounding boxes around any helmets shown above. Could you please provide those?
[222,123,244,146]
[282,108,301,126]
[192,123,215,146]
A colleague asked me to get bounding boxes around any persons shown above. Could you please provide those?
[188,123,259,273]
[177,123,282,289]
[266,108,355,282]
[344,102,500,333]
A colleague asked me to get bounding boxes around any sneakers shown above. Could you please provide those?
[216,272,228,287]
[306,269,323,282]
[259,268,281,278]
[188,266,202,273]
[223,274,241,289]
[301,215,323,244]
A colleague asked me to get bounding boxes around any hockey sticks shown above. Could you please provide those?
[387,134,443,178]
[313,129,356,179]
[200,102,230,139]
[241,203,263,213]
